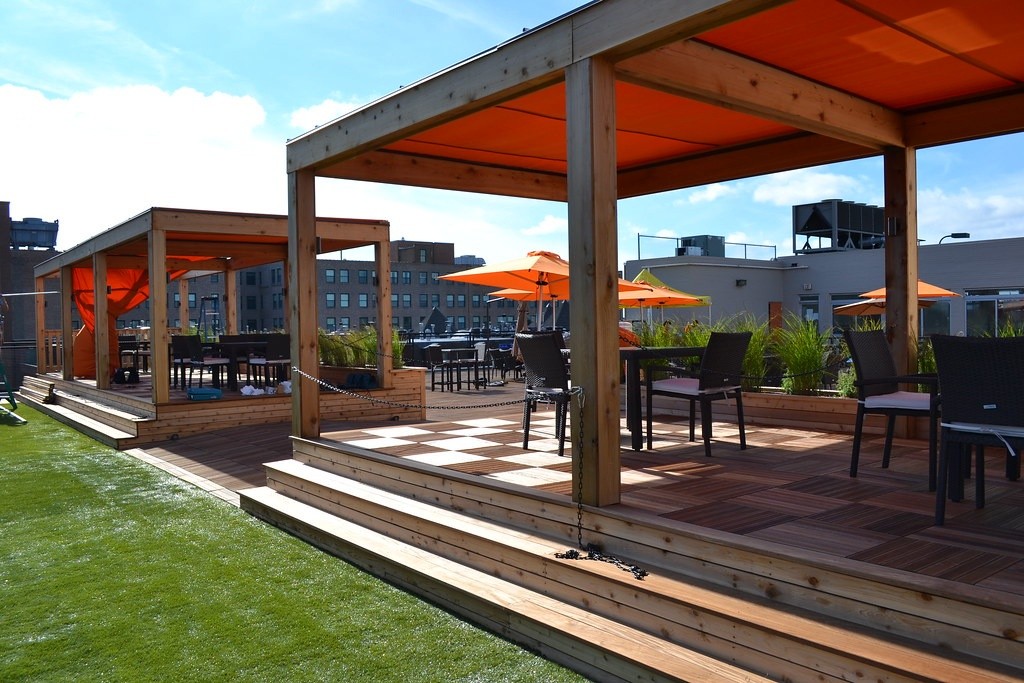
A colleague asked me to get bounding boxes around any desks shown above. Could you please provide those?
[560,345,706,453]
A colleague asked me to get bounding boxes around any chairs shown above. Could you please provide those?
[116,328,520,396]
[521,329,568,427]
[653,332,753,455]
[515,332,571,456]
[844,329,941,492]
[931,334,1023,526]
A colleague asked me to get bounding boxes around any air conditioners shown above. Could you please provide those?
[676,247,702,256]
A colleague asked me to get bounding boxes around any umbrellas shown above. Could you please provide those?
[858,277,961,299]
[832,296,938,315]
[437,251,653,334]
[614,279,704,334]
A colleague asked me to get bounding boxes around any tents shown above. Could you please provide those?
[617,268,712,347]
